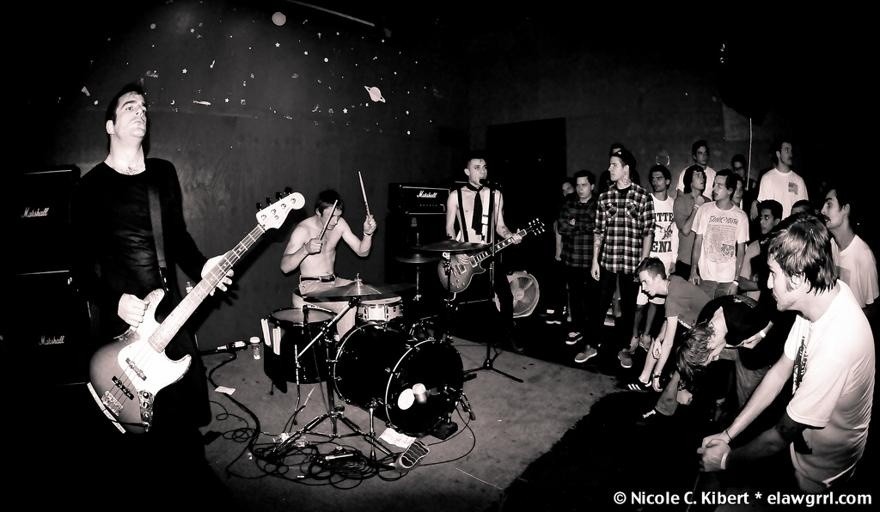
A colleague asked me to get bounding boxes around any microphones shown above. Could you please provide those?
[477,176,500,190]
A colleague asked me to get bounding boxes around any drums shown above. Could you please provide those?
[332,319,464,438]
[358,297,403,325]
[268,308,338,382]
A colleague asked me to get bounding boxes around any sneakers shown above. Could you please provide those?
[616,377,653,392]
[617,333,655,369]
[565,331,583,345]
[574,344,598,363]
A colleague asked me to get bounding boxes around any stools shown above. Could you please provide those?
[292,289,313,309]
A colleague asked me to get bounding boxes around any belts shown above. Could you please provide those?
[300,274,336,283]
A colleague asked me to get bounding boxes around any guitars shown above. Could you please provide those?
[87,186,306,435]
[438,218,547,294]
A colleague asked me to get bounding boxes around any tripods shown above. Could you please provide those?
[461,297,528,387]
[274,350,393,458]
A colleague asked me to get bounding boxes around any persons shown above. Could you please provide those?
[279,188,377,340]
[444,153,525,352]
[55,83,234,512]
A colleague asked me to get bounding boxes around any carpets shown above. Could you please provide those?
[198,323,623,512]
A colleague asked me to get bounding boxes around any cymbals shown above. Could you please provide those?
[416,238,489,253]
[394,254,436,264]
[306,281,417,300]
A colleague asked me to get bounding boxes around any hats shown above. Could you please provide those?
[613,149,640,179]
